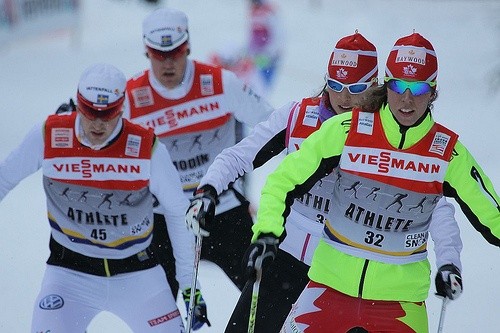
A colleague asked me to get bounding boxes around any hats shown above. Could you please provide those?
[328,29,378,86]
[385,32,438,89]
[142,7,189,51]
[78,63,127,104]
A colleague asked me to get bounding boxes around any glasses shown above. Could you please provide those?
[77,93,125,122]
[383,78,436,96]
[325,74,376,95]
[145,42,188,61]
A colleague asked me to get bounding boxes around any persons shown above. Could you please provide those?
[244,28,500,333]
[185,29,463,333]
[0,63,212,333]
[54,10,288,312]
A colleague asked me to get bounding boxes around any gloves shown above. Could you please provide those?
[435,263,464,299]
[182,288,211,331]
[185,184,220,239]
[245,237,279,282]
